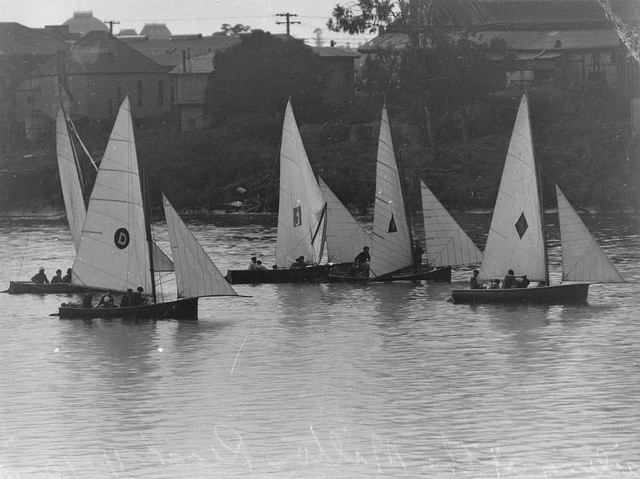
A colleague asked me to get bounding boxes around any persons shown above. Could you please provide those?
[290,259,302,281]
[62,268,71,283]
[99,296,114,307]
[120,289,139,307]
[248,257,258,270]
[504,270,517,288]
[97,290,114,307]
[469,270,487,289]
[414,239,424,271]
[355,246,370,277]
[83,290,93,307]
[298,256,306,281]
[272,264,277,270]
[489,279,500,288]
[256,261,268,270]
[51,269,62,283]
[31,266,49,284]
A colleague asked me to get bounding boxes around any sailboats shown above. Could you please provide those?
[0,97,176,294]
[48,92,253,322]
[327,97,484,283]
[450,91,628,304]
[223,95,372,285]
